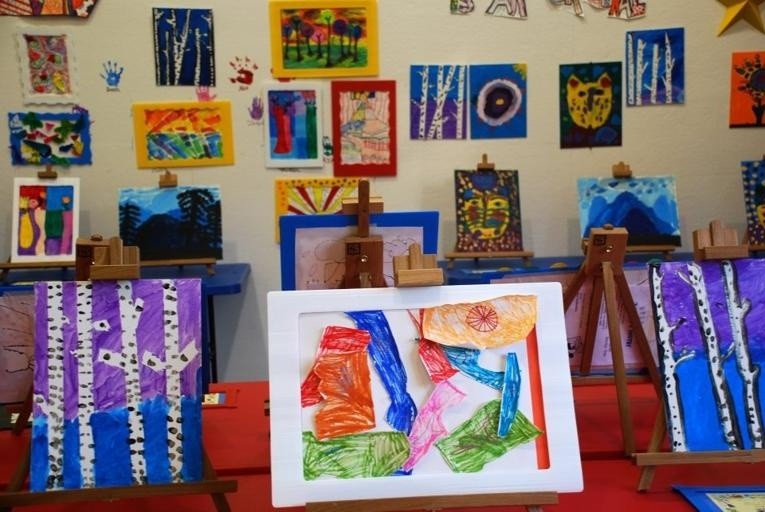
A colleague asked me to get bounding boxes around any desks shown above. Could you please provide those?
[1,262,251,386]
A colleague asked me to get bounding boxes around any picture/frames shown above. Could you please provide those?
[328,78,398,179]
[8,108,94,168]
[264,282,587,508]
[9,175,83,266]
[127,98,238,169]
[148,4,220,90]
[113,184,227,260]
[266,2,383,79]
[13,21,82,107]
[270,176,368,246]
[262,82,328,170]
